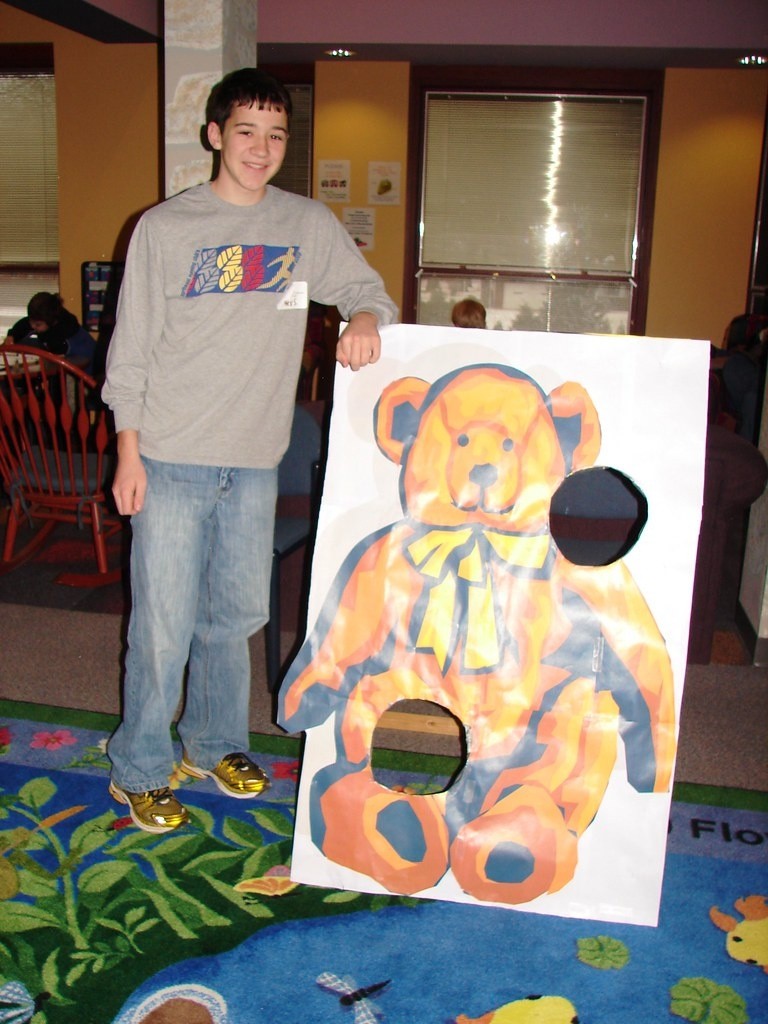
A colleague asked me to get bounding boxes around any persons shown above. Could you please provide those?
[5,292,104,382]
[452,299,486,329]
[101,68,398,834]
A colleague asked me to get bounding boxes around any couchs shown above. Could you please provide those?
[550,422,768,666]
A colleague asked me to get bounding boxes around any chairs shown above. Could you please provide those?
[0,344,133,573]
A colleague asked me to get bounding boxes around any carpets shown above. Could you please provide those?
[0,696,768,1024]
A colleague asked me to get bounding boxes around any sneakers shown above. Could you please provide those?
[181,747,272,799]
[109,777,190,833]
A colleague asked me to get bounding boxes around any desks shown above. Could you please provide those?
[0,352,90,451]
